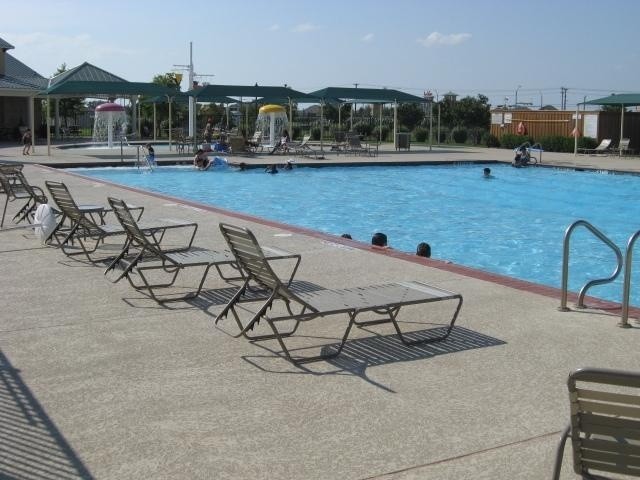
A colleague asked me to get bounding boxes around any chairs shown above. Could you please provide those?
[0,161,200,264]
[210,219,464,365]
[577,137,637,160]
[551,369,640,479]
[166,120,383,163]
[100,195,305,310]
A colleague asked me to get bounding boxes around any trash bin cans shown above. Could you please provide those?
[395,133,410,151]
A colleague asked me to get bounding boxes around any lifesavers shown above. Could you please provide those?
[516,126,525,136]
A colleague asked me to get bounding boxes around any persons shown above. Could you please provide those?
[417,242,452,265]
[21,128,31,154]
[515,146,531,163]
[482,166,493,179]
[145,143,156,163]
[513,155,523,167]
[341,233,353,239]
[194,118,293,175]
[370,233,392,250]
